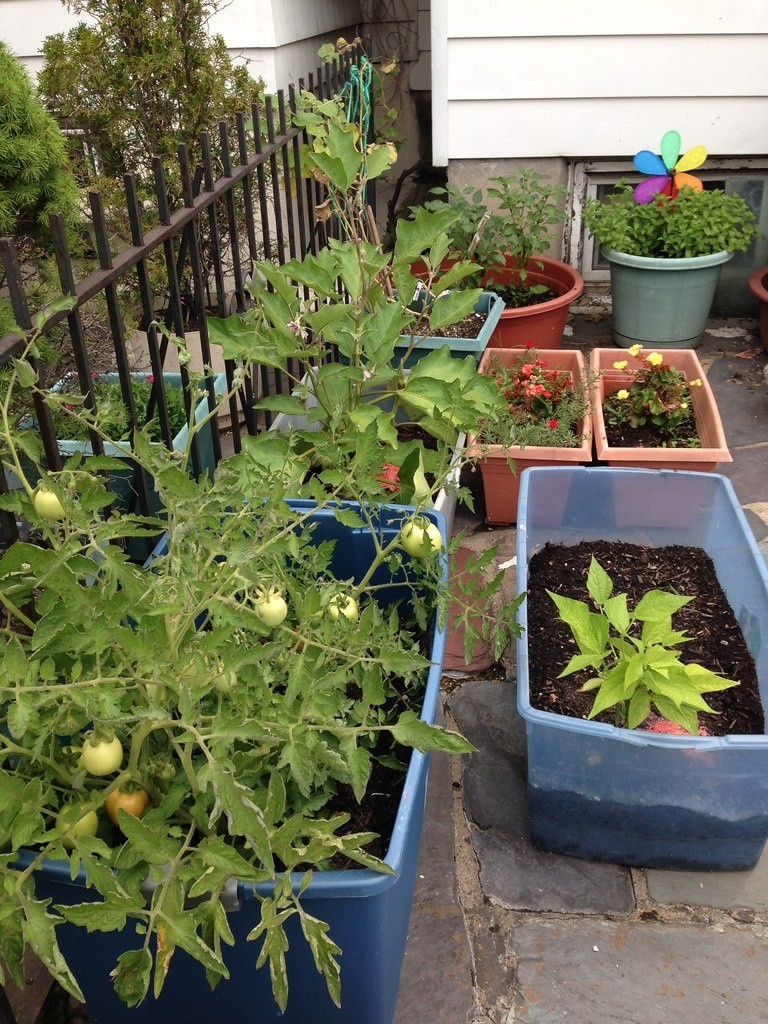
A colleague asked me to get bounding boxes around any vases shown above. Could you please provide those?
[748,266,768,350]
[589,349,734,527]
[465,348,592,529]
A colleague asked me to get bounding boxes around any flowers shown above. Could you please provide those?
[52,370,198,444]
[480,330,606,447]
[603,343,702,435]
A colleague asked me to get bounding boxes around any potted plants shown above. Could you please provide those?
[577,176,764,348]
[373,167,584,350]
